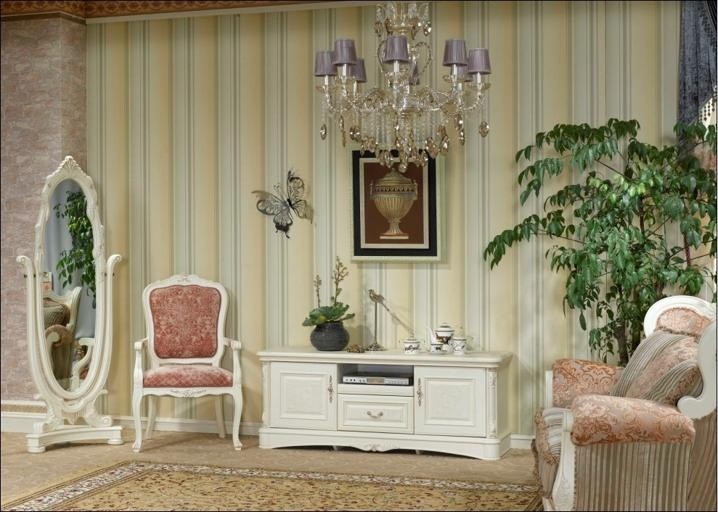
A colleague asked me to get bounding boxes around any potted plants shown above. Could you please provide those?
[302,255,356,352]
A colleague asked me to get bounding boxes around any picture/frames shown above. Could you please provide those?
[346,142,443,264]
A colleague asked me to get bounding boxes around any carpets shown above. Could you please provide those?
[3,452,544,512]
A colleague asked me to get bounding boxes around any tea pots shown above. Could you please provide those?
[429,322,455,353]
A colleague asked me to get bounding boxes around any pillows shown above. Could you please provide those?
[606,326,701,407]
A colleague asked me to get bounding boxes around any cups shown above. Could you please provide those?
[429,343,443,354]
[452,337,467,356]
[404,341,420,354]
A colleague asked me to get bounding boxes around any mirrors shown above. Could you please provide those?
[18,154,124,452]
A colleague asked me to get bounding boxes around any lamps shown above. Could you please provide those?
[313,0,495,177]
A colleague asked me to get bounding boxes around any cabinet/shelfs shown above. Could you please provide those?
[254,350,515,462]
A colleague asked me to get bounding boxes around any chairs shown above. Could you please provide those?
[129,271,247,455]
[527,294,717,512]
